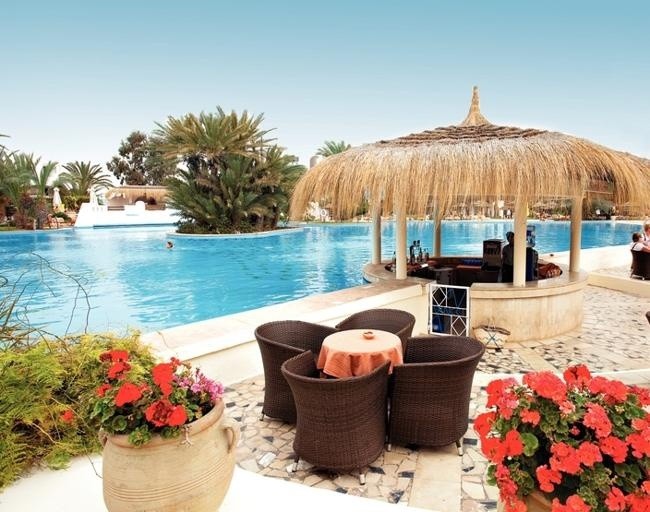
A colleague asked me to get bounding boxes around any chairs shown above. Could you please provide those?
[335,309,416,354]
[281,350,391,484]
[255,320,338,422]
[629,249,650,281]
[386,336,485,456]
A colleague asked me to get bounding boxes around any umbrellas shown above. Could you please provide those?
[52,187,62,209]
[90,191,98,209]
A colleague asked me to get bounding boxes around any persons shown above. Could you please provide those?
[500,231,514,282]
[166,241,173,248]
[632,223,650,253]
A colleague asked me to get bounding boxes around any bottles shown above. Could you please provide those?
[409,240,430,265]
[392,251,397,273]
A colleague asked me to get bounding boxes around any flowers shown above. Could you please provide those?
[471,362,650,512]
[63,350,225,449]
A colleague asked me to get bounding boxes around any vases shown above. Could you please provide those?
[100,393,239,511]
